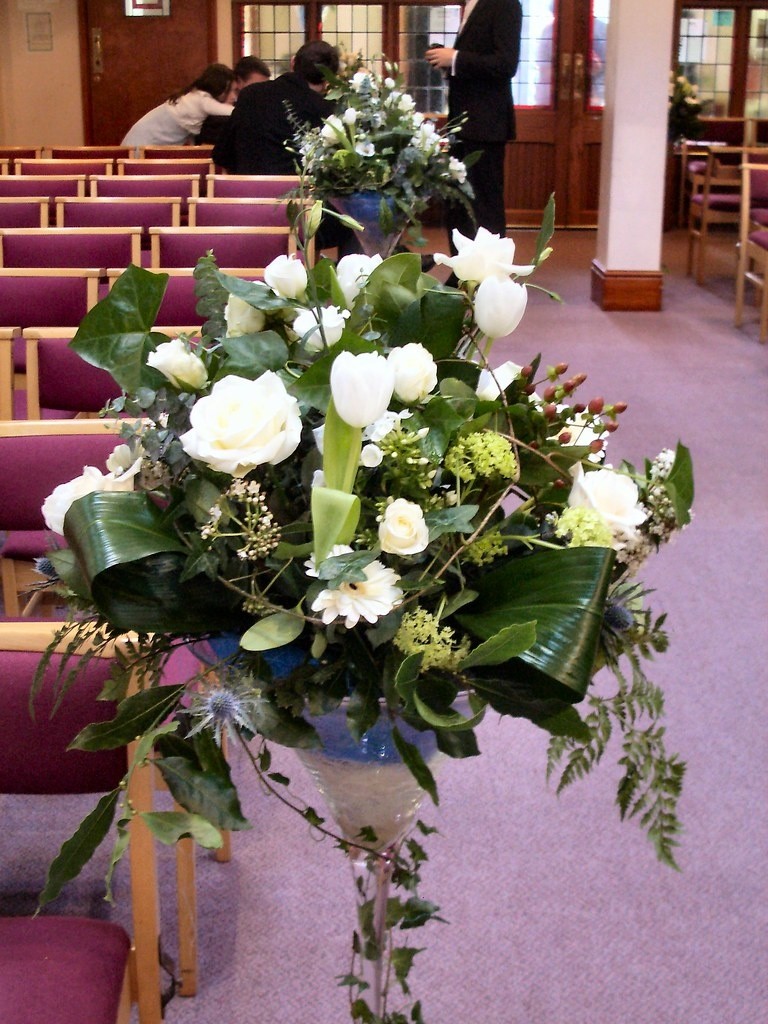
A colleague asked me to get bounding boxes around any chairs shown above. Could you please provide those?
[0,144,321,1024]
[678,143,768,347]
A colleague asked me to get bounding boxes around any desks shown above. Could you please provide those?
[673,145,709,226]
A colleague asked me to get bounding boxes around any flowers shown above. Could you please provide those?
[281,42,481,255]
[15,184,693,1024]
[667,66,713,147]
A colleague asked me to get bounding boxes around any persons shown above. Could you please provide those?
[211,41,337,175]
[200,56,270,144]
[120,64,236,146]
[424,0,522,255]
[536,0,607,106]
